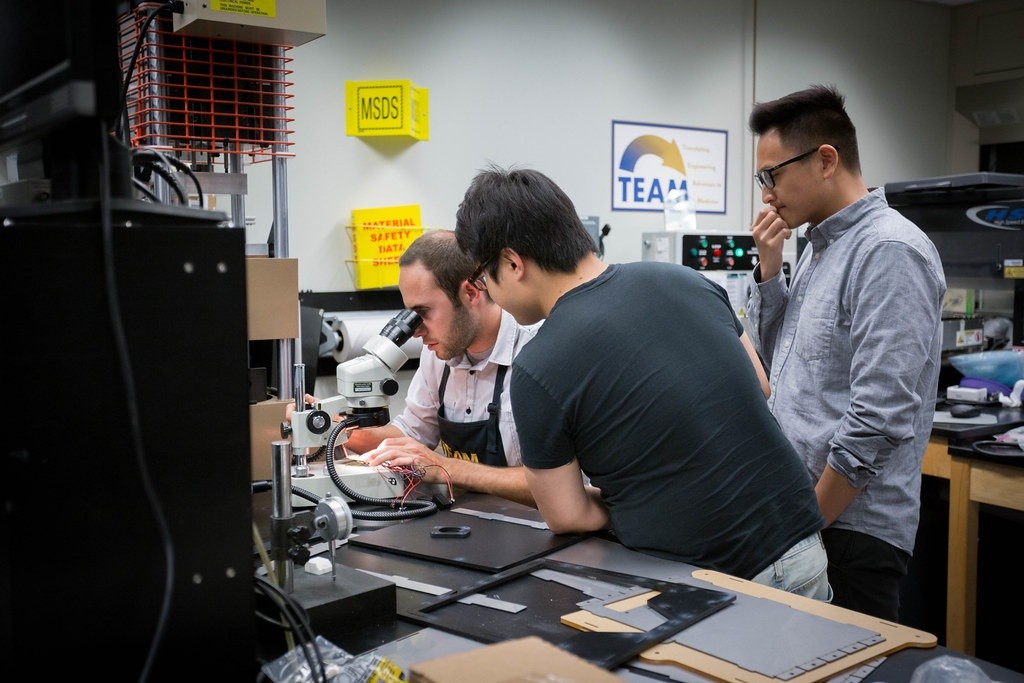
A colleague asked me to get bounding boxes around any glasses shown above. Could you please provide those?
[468,245,521,291]
[755,145,841,190]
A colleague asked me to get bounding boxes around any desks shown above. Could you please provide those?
[322,479,1024,683]
[920,422,1024,656]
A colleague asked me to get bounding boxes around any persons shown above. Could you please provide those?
[456,169,834,606]
[742,85,949,623]
[285,228,539,502]
